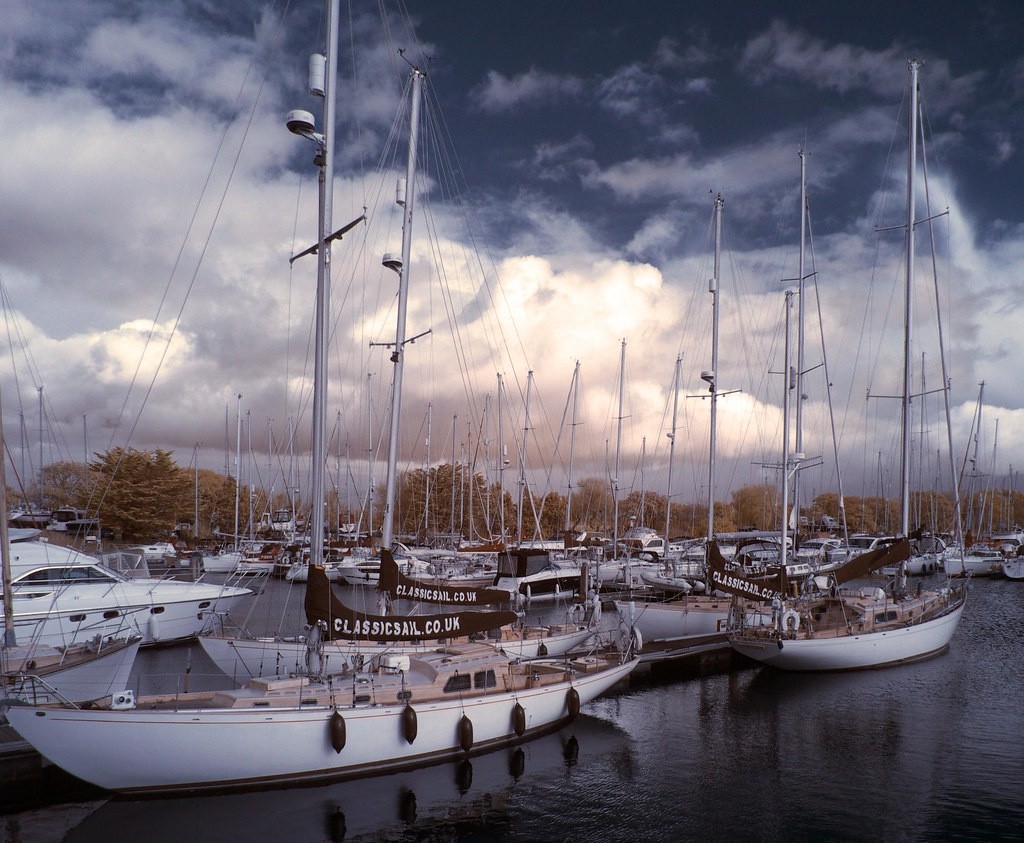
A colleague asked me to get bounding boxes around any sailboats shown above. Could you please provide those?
[1,0,1024,791]
